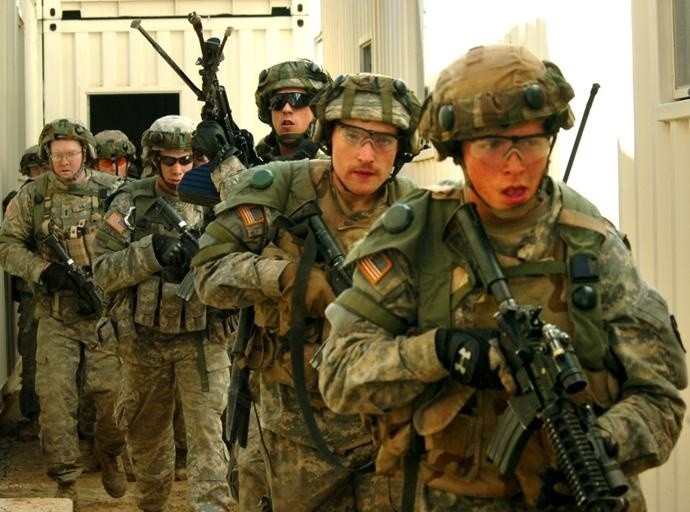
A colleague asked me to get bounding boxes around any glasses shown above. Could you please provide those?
[157,156,195,167]
[48,151,83,162]
[267,92,311,111]
[100,157,126,168]
[466,133,550,162]
[338,122,401,153]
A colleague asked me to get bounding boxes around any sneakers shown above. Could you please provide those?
[1,393,230,506]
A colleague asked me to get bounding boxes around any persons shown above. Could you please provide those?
[2,144,51,443]
[190,59,334,511]
[308,42,690,512]
[172,392,188,480]
[191,74,427,512]
[1,118,126,512]
[91,115,231,511]
[76,130,137,474]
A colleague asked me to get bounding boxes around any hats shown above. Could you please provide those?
[141,114,198,166]
[94,130,137,159]
[19,145,48,176]
[418,44,575,164]
[38,118,97,167]
[300,72,430,167]
[255,58,332,124]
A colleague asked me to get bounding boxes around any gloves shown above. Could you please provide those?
[277,262,337,319]
[152,233,191,280]
[537,466,629,511]
[40,261,78,294]
[73,280,102,321]
[435,328,506,389]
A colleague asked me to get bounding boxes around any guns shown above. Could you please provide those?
[132,13,254,169]
[145,198,199,302]
[296,204,352,297]
[40,236,103,316]
[443,202,630,507]
